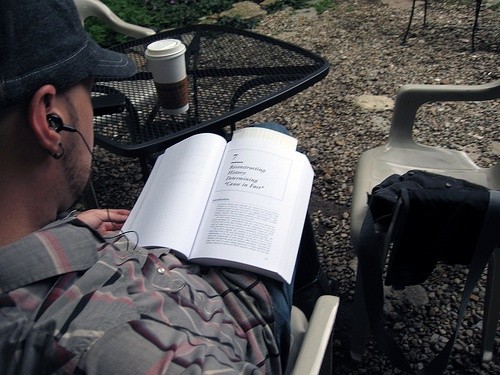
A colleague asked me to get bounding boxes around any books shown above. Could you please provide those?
[118,128,315,285]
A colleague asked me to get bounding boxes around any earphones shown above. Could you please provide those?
[46,113,76,134]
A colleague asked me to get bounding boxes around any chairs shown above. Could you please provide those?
[283,295,340,375]
[73,0,158,129]
[352,80,500,361]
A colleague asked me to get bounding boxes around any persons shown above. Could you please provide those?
[0,1,292,374]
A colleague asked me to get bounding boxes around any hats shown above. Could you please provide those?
[0,0,137,106]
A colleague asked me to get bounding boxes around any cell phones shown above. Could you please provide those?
[91,93,126,116]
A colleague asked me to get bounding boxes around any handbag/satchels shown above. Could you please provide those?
[358,170,500,375]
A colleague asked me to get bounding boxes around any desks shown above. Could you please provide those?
[93,23,331,186]
